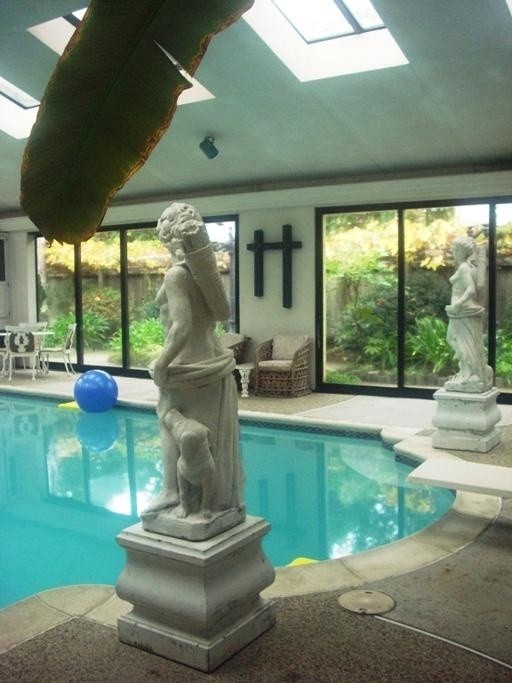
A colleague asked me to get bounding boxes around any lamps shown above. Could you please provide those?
[0,321,78,381]
[218,332,314,397]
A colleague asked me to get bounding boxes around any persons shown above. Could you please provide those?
[443,236,491,383]
[141,200,244,519]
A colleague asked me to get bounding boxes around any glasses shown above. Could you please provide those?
[199,134,219,160]
